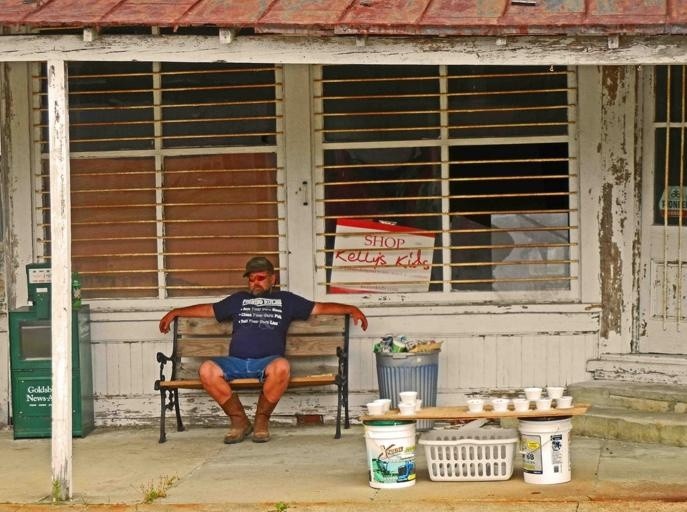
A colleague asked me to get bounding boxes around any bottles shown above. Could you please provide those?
[72,271,82,307]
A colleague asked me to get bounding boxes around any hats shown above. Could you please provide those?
[243,257,273,278]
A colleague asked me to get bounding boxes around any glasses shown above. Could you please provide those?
[248,275,269,282]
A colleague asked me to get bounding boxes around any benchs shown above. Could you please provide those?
[153,315,349,443]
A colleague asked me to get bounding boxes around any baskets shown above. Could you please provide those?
[418,429,517,482]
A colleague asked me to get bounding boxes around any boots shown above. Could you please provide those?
[253,390,280,442]
[221,392,252,443]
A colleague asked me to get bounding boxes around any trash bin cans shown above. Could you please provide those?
[375,350,438,429]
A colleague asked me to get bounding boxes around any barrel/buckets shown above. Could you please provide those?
[363,420,417,489]
[518,418,574,485]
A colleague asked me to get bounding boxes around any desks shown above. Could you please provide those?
[359,402,590,421]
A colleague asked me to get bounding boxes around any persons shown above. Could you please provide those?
[157,256,368,443]
